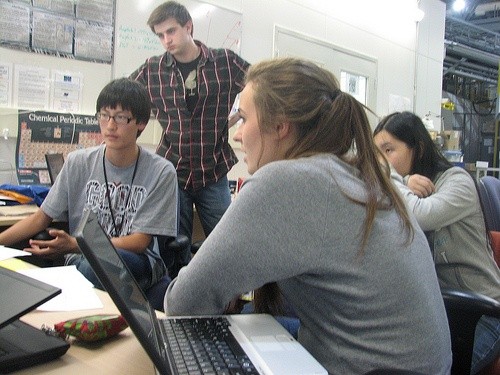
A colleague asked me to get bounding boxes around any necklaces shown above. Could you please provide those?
[101,143,141,239]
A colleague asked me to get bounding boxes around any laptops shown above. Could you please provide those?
[45,153,64,185]
[74,208,329,375]
[0,265,71,372]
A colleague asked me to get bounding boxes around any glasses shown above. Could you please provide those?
[95,112,138,125]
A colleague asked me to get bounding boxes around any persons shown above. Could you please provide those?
[162,57,454,375]
[0,76,179,314]
[121,0,254,285]
[368,110,500,375]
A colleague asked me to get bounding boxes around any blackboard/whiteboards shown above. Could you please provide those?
[112,0,243,121]
[273,25,378,135]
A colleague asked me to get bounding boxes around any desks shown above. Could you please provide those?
[0,203,165,375]
